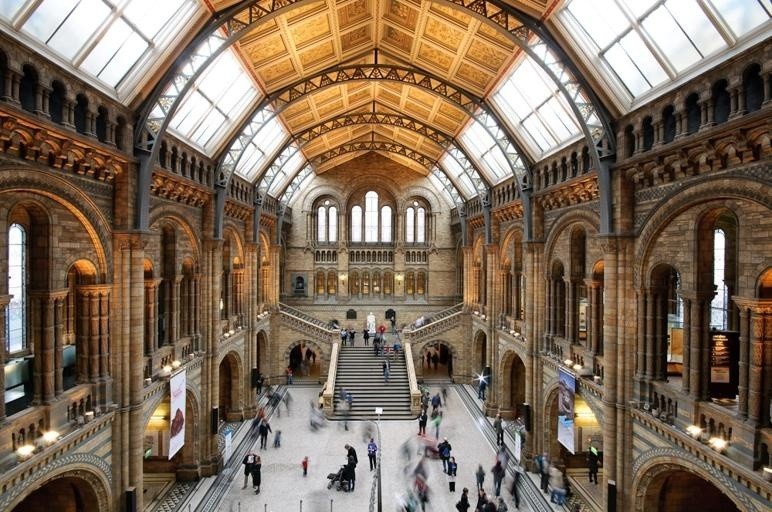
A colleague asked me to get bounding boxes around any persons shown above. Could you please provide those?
[587,445,601,486]
[302,456,310,478]
[420,313,427,327]
[248,346,326,451]
[241,450,256,491]
[414,314,423,329]
[382,357,391,383]
[344,444,358,465]
[366,311,377,335]
[340,325,401,360]
[252,455,262,496]
[345,456,356,492]
[367,438,377,472]
[391,317,397,335]
[396,347,573,511]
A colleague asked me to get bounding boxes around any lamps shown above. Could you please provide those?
[398,273,401,285]
[341,272,345,286]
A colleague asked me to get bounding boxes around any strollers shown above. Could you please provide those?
[326,464,352,492]
